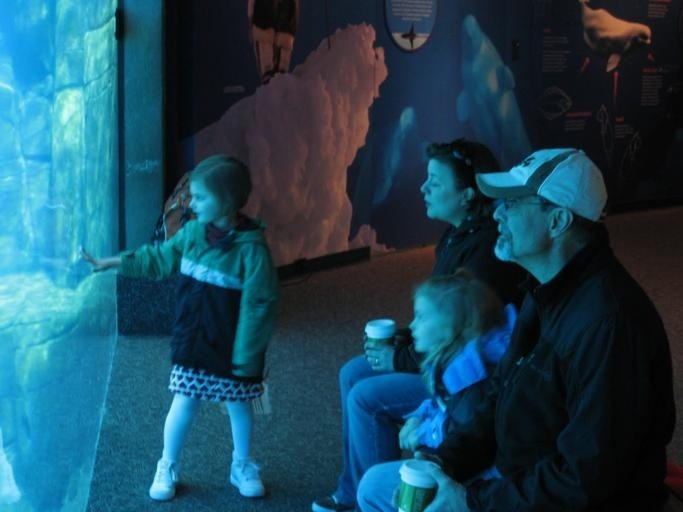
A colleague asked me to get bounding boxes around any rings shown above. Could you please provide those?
[375,358,379,365]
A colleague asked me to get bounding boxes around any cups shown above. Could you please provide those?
[396,460,441,511]
[364,319,395,346]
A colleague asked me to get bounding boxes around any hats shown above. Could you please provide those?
[475,150,607,221]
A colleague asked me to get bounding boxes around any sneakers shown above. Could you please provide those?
[149,458,179,501]
[311,494,353,511]
[229,450,265,496]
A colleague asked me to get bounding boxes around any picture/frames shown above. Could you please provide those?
[383,0,438,53]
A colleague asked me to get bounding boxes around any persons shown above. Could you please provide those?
[313,137,536,512]
[357,148,678,512]
[79,155,278,501]
[399,267,518,449]
